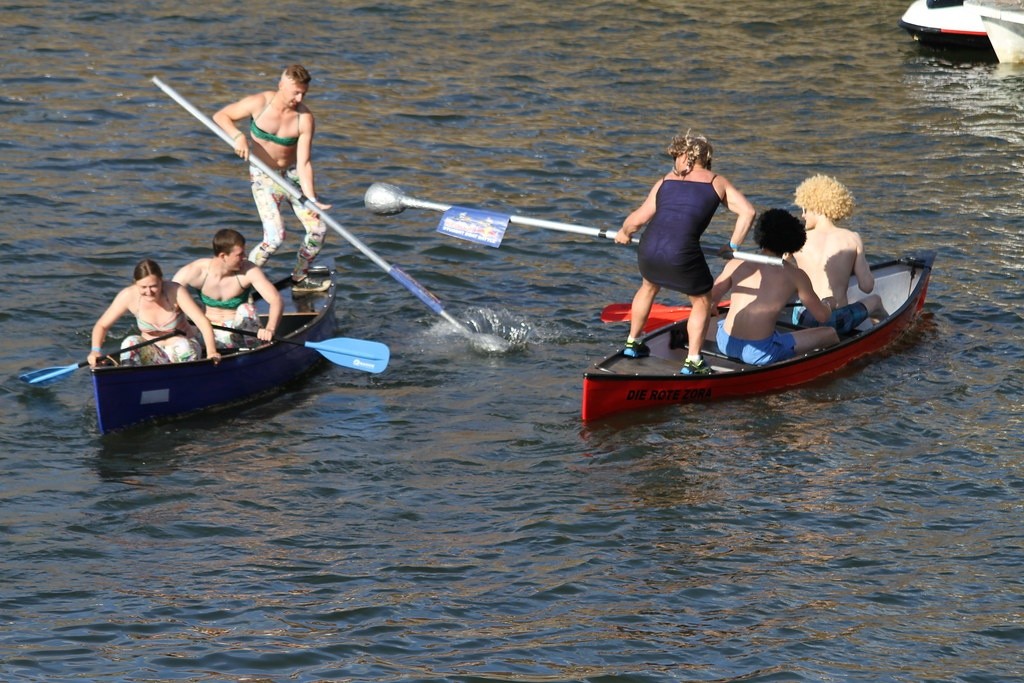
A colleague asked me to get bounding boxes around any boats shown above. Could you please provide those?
[90,256,339,434]
[581,248,938,422]
[899,2,1024,59]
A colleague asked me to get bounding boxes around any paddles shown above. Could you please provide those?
[601,301,803,335]
[188,320,390,373]
[20,327,187,384]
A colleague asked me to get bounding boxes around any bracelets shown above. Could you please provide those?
[309,197,317,203]
[233,132,243,140]
[728,241,740,249]
[91,347,102,351]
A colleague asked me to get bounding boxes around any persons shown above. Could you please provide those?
[87,259,222,366]
[615,135,756,375]
[213,64,331,292]
[782,174,888,337]
[171,229,284,349]
[709,209,841,366]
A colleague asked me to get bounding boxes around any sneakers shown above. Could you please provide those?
[292,275,331,291]
[623,340,652,358]
[681,357,712,375]
